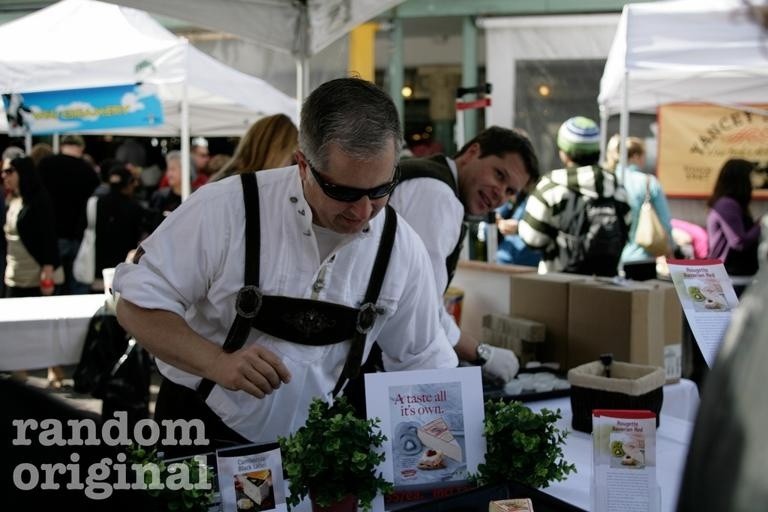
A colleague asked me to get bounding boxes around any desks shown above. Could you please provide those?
[457,345,705,512]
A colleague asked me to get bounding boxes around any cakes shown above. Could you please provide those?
[418,449,442,468]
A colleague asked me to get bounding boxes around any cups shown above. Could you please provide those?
[101,267,115,299]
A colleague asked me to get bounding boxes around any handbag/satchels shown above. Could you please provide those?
[634,201,673,256]
[560,196,632,276]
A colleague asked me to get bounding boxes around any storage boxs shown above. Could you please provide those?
[506,271,578,336]
[570,357,665,429]
[569,274,684,380]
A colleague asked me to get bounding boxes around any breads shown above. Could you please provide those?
[700,283,726,308]
[241,476,268,506]
[418,416,463,463]
[623,441,644,465]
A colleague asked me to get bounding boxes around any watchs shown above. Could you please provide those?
[476,341,506,368]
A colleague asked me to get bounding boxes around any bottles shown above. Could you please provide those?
[443,288,463,326]
[39,265,55,296]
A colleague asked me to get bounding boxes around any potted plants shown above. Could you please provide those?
[277,398,388,512]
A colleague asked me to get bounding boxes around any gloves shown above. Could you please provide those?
[477,344,520,382]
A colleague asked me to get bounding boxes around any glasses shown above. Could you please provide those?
[306,160,401,203]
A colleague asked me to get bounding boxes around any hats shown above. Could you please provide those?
[557,116,601,155]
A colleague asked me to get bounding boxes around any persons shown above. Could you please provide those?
[389,128,539,385]
[0,113,762,294]
[100,77,460,445]
